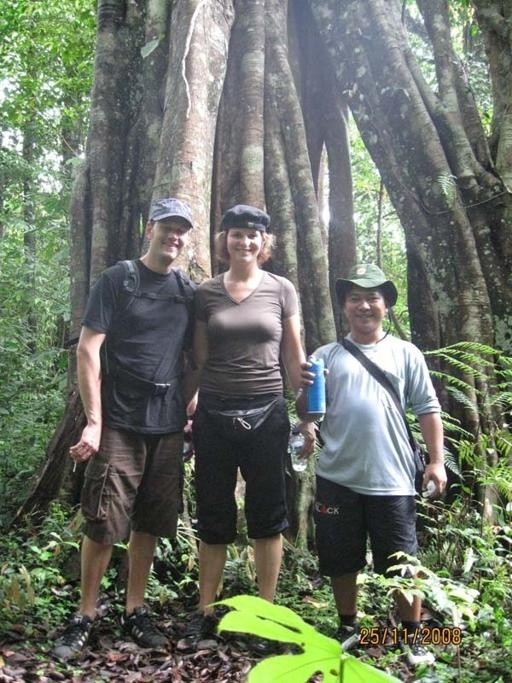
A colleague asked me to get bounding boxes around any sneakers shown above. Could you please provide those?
[175,612,219,654]
[398,624,437,666]
[330,619,362,650]
[119,605,169,649]
[250,631,281,658]
[51,610,98,661]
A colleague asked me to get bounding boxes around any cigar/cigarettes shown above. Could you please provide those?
[72,460,77,473]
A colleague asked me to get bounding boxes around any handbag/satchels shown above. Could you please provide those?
[410,435,430,497]
[205,401,273,446]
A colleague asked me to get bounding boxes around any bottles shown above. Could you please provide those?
[307,357,326,416]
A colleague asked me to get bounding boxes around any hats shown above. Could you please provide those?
[150,197,195,227]
[219,204,272,233]
[335,263,397,308]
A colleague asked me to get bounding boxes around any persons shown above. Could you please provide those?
[192,203,316,618]
[54,198,196,662]
[294,264,448,664]
